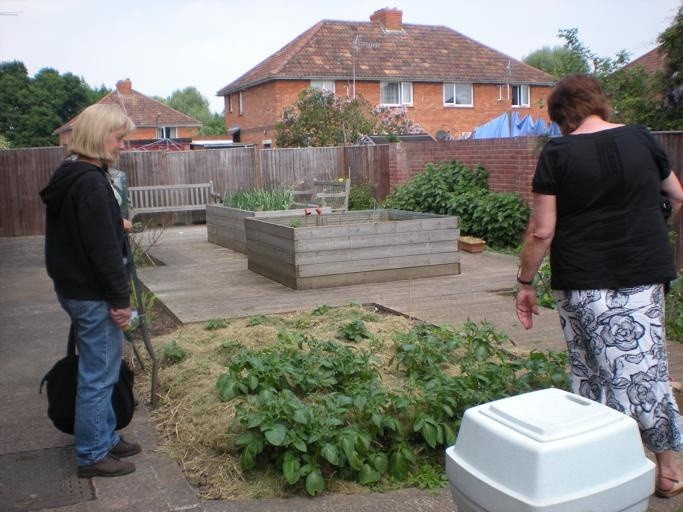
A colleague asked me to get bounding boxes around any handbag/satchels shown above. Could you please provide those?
[37,313,137,433]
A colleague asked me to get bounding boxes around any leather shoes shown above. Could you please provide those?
[106,437,140,457]
[77,455,137,478]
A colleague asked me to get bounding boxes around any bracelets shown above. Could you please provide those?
[516,277,534,285]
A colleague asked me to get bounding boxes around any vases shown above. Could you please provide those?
[459,236,485,253]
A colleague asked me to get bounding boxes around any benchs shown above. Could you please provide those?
[286,181,349,213]
[124,182,222,227]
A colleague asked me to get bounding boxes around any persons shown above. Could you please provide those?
[44,103,141,478]
[64,153,139,408]
[512,72,683,499]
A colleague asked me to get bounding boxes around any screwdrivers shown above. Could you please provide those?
[122,324,146,371]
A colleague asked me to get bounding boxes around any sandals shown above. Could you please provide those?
[656,475,683,497]
